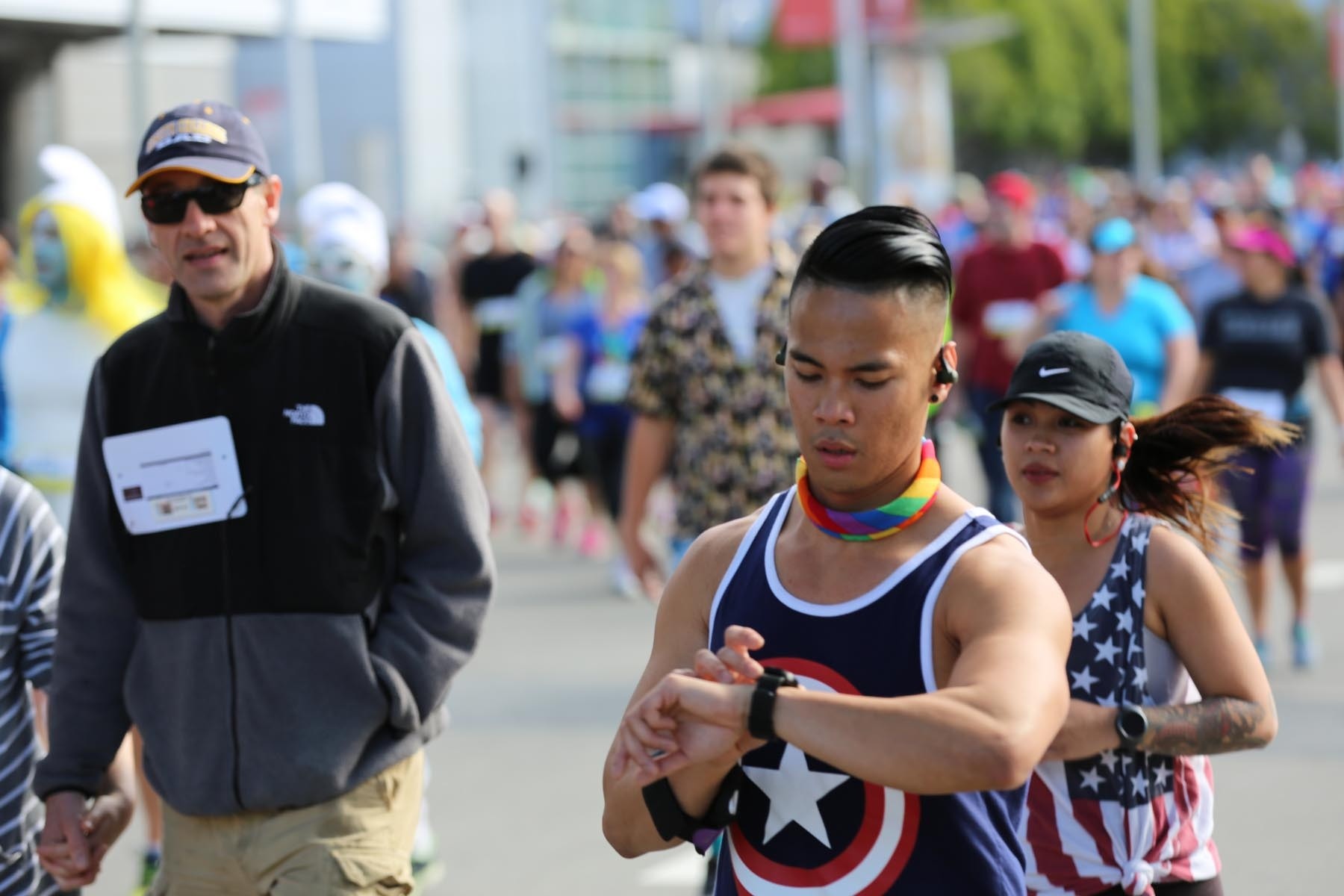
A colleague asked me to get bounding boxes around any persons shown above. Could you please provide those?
[282,179,490,890]
[2,453,139,894]
[28,99,499,895]
[1188,207,1341,671]
[3,140,1343,597]
[593,202,1079,896]
[972,315,1278,896]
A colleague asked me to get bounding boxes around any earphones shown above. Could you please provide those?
[936,357,959,385]
[774,342,787,366]
[1113,441,1127,459]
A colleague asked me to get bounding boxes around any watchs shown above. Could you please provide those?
[747,658,798,741]
[1111,700,1152,758]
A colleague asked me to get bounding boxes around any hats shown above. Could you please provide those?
[124,101,272,197]
[987,329,1134,424]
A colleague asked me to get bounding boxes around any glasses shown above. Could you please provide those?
[141,172,262,226]
